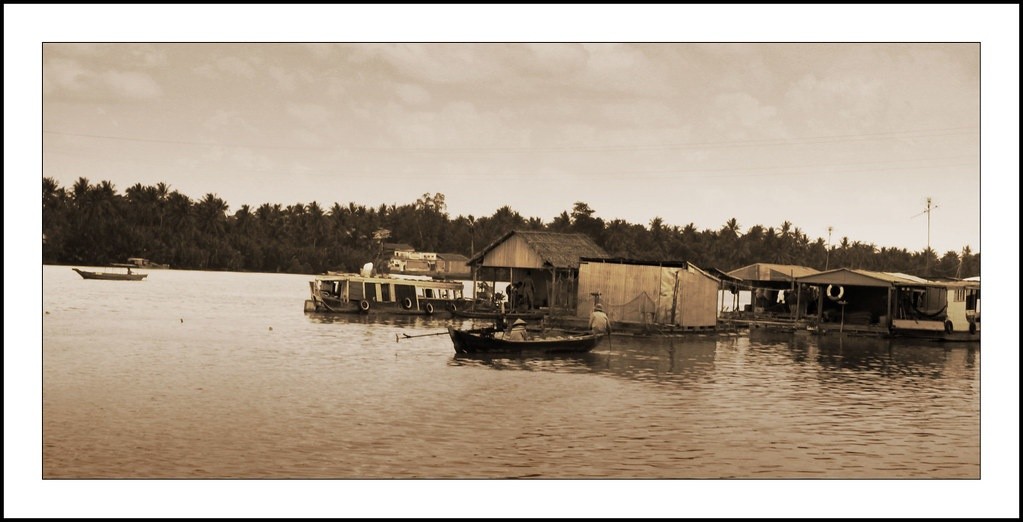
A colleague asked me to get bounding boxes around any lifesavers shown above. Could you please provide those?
[969,322,976,334]
[944,320,954,334]
[359,299,370,312]
[402,296,412,310]
[826,284,845,301]
[446,302,457,313]
[425,302,434,314]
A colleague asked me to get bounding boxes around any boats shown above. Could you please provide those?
[748,322,796,334]
[72,267,148,281]
[445,302,545,320]
[446,324,606,356]
[110,258,170,269]
[303,270,464,315]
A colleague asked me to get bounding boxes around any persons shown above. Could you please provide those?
[786,292,797,312]
[509,318,528,341]
[127,267,131,274]
[522,272,536,310]
[506,281,522,302]
[588,304,611,334]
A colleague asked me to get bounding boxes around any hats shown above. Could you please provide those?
[512,318,528,326]
[593,303,605,311]
